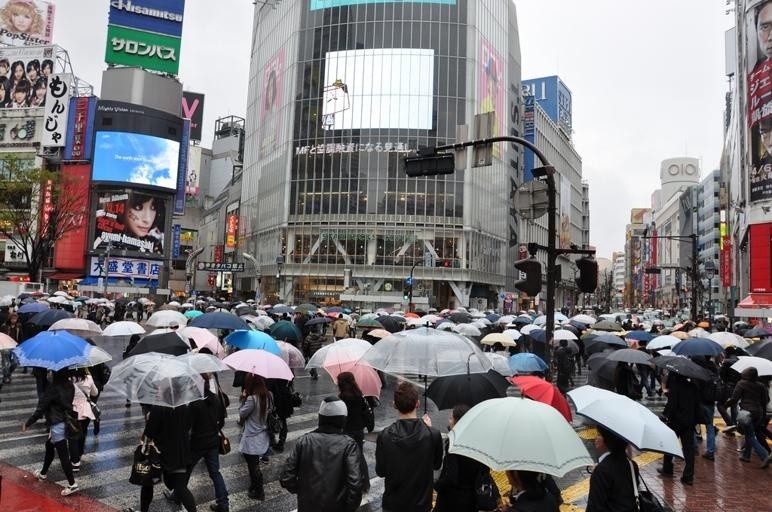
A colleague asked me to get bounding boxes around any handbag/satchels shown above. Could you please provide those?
[268,410,281,434]
[217,430,231,455]
[476,473,506,512]
[736,410,752,426]
[291,390,303,407]
[128,440,162,485]
[636,487,673,512]
[89,401,101,418]
[364,400,375,433]
[696,402,712,424]
[65,411,83,440]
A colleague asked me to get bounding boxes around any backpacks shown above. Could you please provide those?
[95,362,111,386]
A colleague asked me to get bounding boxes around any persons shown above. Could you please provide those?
[750,114,771,202]
[1,59,54,109]
[749,1,772,72]
[0,379,768,511]
[480,56,502,160]
[1,0,47,47]
[96,192,166,255]
[259,71,278,160]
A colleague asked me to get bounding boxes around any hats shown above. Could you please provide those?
[317,395,350,427]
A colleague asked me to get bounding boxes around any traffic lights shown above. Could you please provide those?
[403,291,409,300]
[513,258,541,298]
[576,255,597,294]
[434,259,450,267]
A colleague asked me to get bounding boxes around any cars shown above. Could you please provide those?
[617,302,676,320]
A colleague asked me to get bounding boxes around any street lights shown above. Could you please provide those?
[274,252,285,305]
[704,260,715,334]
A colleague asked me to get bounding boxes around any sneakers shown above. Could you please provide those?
[210,503,229,512]
[33,469,48,482]
[61,483,80,496]
[164,488,180,504]
[759,456,771,468]
[248,491,266,501]
[739,456,750,462]
[722,425,736,434]
[702,452,715,460]
[680,477,693,485]
[71,461,80,472]
[656,467,673,477]
